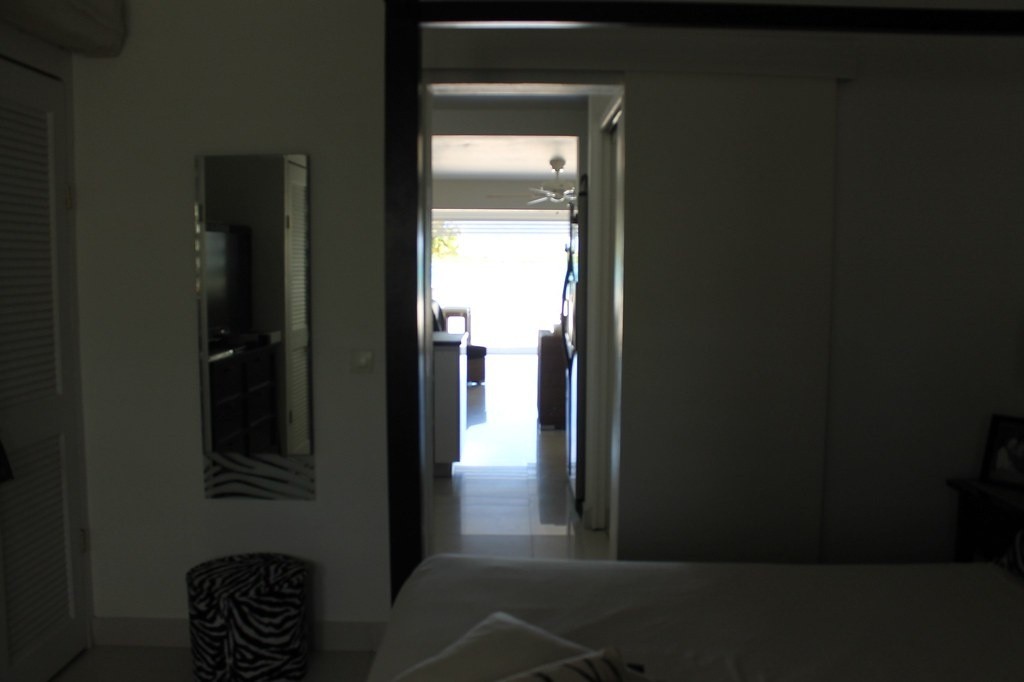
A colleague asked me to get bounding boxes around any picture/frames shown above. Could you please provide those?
[979,414,1024,492]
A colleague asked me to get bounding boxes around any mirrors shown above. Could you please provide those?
[193,153,315,500]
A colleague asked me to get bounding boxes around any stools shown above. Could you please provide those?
[185,553,310,682]
[467,345,486,385]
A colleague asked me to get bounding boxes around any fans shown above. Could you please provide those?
[527,158,577,204]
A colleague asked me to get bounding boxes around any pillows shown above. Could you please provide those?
[391,612,651,682]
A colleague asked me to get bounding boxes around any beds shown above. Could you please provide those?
[368,553,1024,682]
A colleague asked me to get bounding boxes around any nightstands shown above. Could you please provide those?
[945,479,1024,576]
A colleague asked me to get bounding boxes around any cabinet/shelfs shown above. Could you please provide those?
[209,343,281,457]
[432,331,469,477]
[0,19,93,682]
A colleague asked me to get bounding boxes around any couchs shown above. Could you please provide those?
[537,330,567,430]
